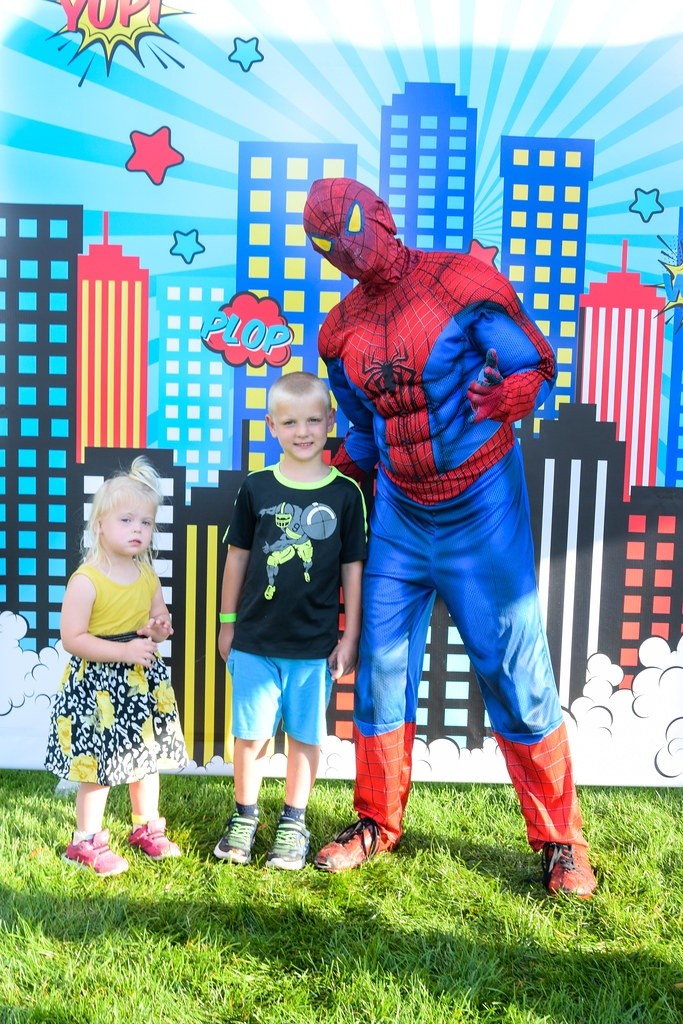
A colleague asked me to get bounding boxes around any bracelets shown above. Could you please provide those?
[219,612,236,623]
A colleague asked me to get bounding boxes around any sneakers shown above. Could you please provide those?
[265,811,310,870]
[213,811,260,864]
[61,828,127,876]
[128,817,181,860]
[541,841,598,899]
[313,816,398,873]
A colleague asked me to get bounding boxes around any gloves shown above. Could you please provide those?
[465,349,503,425]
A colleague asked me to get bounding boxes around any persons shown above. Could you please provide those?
[43,452,188,878]
[213,372,370,870]
[303,177,599,902]
